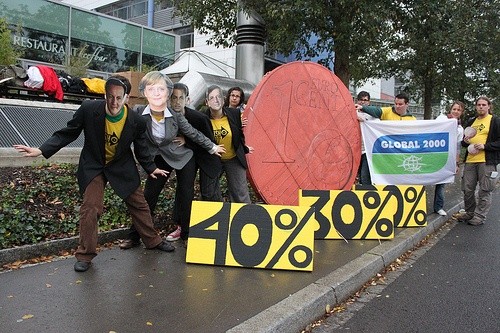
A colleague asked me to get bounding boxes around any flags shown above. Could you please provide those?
[358,116,458,188]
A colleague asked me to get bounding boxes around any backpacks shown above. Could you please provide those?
[54,68,88,94]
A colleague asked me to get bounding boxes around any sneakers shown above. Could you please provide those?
[167,225,184,241]
[436,209,447,216]
[468,215,485,225]
[458,212,472,221]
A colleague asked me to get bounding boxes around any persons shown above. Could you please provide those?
[118,72,227,253]
[454,96,500,225]
[198,85,255,204]
[165,83,225,241]
[433,100,464,216]
[14,75,176,271]
[221,85,248,130]
[354,92,418,122]
[354,90,380,186]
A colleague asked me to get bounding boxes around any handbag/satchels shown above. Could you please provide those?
[24,65,45,88]
[1,64,29,86]
[79,77,107,95]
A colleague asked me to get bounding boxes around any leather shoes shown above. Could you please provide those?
[154,241,176,251]
[75,260,90,271]
[120,239,141,249]
